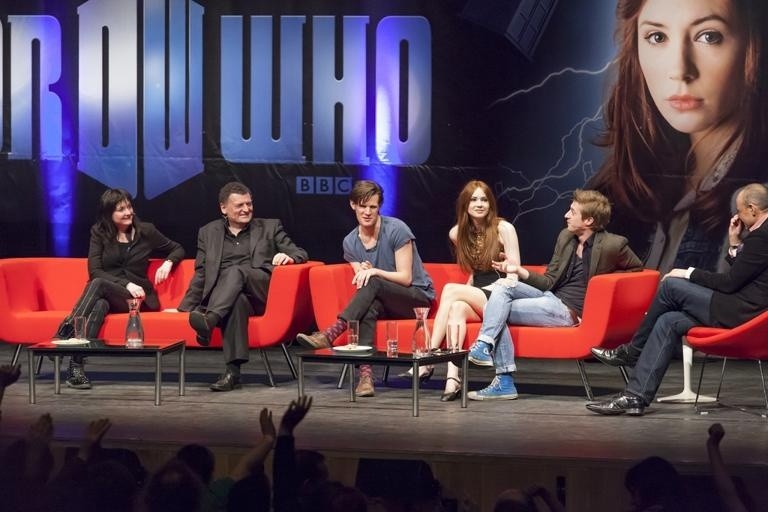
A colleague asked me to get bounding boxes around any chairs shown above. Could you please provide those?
[684,304,767,422]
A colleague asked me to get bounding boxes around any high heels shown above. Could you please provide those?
[397,365,434,384]
[441,377,462,401]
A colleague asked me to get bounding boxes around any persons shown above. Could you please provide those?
[394,180,521,402]
[156,181,311,392]
[586,0,767,283]
[47,188,186,389]
[451,187,644,402]
[586,182,768,416]
[295,178,436,397]
[3,392,747,510]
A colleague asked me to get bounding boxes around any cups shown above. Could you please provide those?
[385,320,398,355]
[346,320,359,351]
[447,322,458,354]
[74,315,85,342]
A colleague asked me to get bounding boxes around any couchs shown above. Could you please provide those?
[307,259,657,405]
[1,257,322,385]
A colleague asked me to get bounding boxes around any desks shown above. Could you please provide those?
[17,336,190,405]
[289,343,474,417]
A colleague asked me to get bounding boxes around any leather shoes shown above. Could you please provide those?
[355,374,375,397]
[585,392,645,416]
[65,362,92,389]
[589,343,639,370]
[210,374,242,392]
[189,312,212,346]
[296,331,331,349]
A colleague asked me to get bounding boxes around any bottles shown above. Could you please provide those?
[124,298,145,349]
[409,307,430,360]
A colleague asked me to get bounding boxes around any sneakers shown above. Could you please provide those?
[466,341,494,366]
[467,373,519,400]
